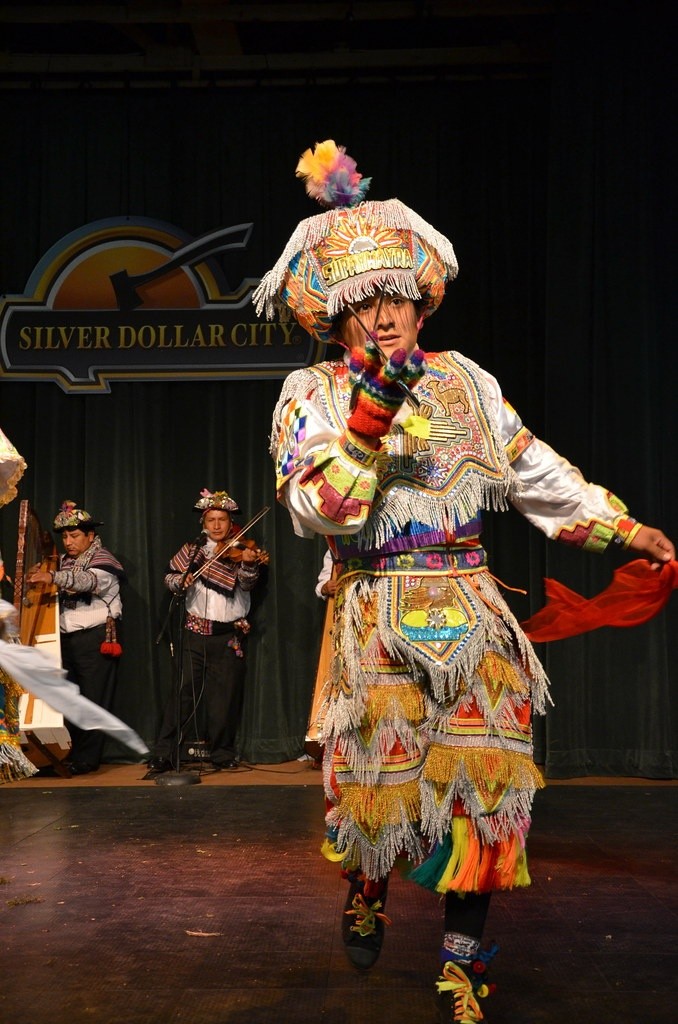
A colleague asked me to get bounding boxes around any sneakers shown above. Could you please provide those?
[433,950,507,1024]
[342,865,388,968]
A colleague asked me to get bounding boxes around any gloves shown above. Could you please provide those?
[346,331,428,438]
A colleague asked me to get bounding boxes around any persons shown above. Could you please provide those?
[23,499,123,773]
[251,138,676,1024]
[147,487,269,770]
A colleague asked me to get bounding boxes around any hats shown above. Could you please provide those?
[251,140,459,342]
[53,501,93,533]
[195,490,238,520]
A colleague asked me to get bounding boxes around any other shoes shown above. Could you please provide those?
[61,755,102,776]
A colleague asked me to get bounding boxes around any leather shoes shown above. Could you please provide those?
[213,756,238,770]
[147,755,172,771]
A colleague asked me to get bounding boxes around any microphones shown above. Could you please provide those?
[193,529,210,546]
[6,575,31,607]
